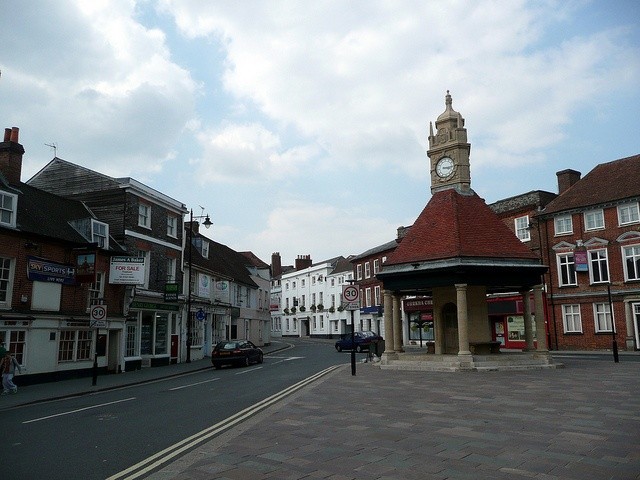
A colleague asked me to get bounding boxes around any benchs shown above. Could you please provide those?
[426,340,434,353]
[470,340,502,355]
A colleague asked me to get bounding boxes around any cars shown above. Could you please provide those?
[335,331,383,352]
[212,340,264,369]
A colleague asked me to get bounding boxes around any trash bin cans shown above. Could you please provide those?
[369,338,384,357]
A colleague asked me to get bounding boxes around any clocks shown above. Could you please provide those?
[434,155,455,180]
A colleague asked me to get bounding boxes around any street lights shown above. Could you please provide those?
[526,220,551,351]
[187,208,213,363]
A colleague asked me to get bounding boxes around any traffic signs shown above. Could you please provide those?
[342,285,360,311]
[90,305,108,328]
